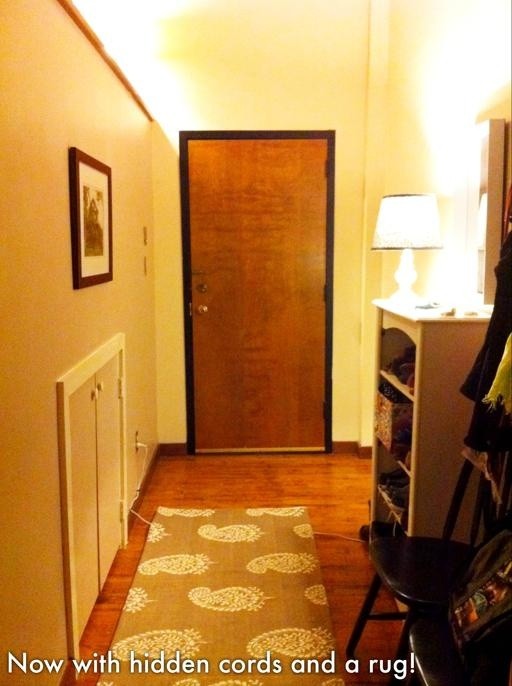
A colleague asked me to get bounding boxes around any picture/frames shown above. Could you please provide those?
[68,146,113,291]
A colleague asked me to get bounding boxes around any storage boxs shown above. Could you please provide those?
[372,390,413,459]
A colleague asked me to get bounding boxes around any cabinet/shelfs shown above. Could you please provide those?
[56,331,130,663]
[362,301,490,549]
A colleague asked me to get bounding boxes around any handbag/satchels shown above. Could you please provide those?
[447,528,511,666]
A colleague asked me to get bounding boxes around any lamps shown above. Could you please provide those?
[369,194,444,307]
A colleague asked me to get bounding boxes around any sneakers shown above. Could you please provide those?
[359,520,394,541]
[389,345,416,389]
[379,467,409,527]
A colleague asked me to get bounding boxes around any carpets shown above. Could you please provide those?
[95,507,345,685]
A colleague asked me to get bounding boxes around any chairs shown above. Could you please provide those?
[345,424,512,685]
